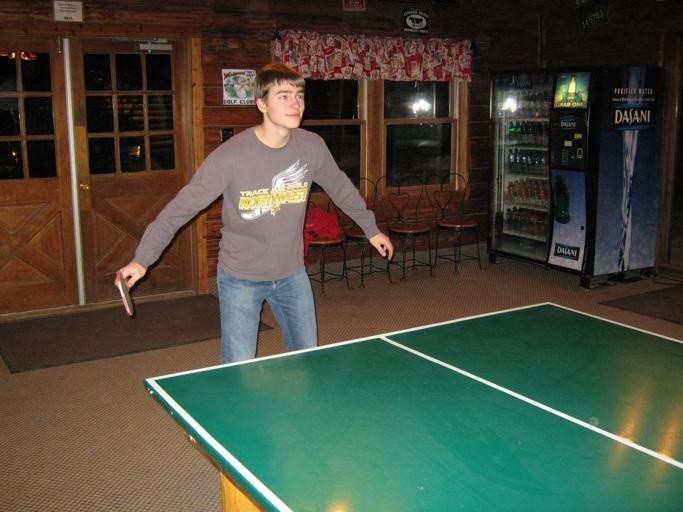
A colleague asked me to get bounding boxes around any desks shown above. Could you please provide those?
[143,302,683,512]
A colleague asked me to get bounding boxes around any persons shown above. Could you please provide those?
[109,63,396,367]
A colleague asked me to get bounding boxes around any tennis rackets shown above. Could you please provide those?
[117,271,133,317]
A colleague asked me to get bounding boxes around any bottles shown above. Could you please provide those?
[502,89,551,240]
[554,175,570,224]
[566,74,576,104]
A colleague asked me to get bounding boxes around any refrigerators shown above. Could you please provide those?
[486,53,664,288]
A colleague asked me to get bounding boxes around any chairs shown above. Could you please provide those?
[303,173,482,298]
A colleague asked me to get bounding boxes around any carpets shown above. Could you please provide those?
[597,283,683,325]
